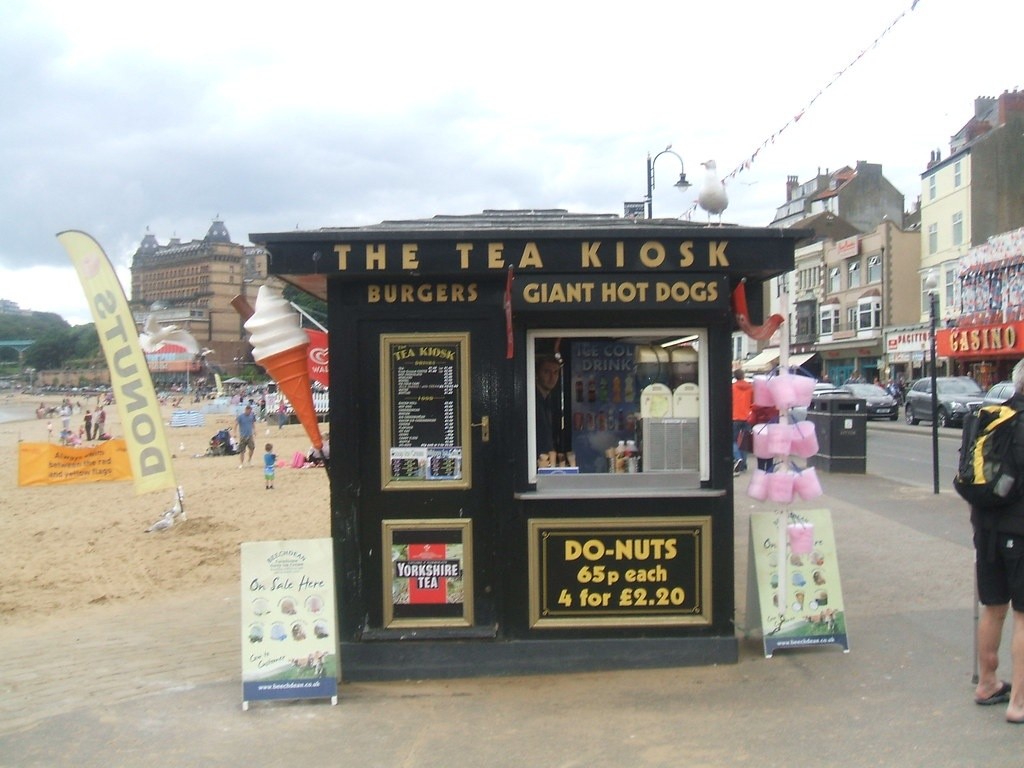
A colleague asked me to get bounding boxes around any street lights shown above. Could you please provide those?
[647,145,693,221]
[924,274,941,495]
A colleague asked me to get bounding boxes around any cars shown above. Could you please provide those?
[812,382,839,396]
[839,382,900,422]
[982,380,1016,409]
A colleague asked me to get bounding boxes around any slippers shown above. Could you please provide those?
[974,680,1013,704]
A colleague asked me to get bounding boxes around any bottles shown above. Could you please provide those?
[618,409,624,430]
[615,440,627,473]
[613,377,621,402]
[625,410,634,431]
[624,377,634,402]
[601,375,608,400]
[608,411,614,432]
[575,373,583,402]
[599,411,605,431]
[588,375,595,402]
[627,440,639,473]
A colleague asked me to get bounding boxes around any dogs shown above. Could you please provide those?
[804,608,838,630]
[289,651,328,677]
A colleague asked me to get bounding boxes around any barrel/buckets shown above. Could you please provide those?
[753,366,780,407]
[790,461,822,500]
[768,416,792,457]
[748,464,775,501]
[766,367,795,411]
[789,514,813,555]
[752,415,778,459]
[792,366,817,408]
[791,414,820,459]
[768,468,794,504]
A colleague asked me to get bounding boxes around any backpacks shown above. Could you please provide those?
[953,399,1024,507]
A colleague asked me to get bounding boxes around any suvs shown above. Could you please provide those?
[904,375,984,430]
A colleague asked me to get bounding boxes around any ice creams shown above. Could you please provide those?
[230,286,322,450]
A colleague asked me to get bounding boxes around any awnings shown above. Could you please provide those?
[745,348,780,365]
[787,352,816,369]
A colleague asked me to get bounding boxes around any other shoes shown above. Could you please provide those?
[239,464,244,469]
[248,461,254,466]
[270,486,273,489]
[266,486,269,489]
[734,459,743,471]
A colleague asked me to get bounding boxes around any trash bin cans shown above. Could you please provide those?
[805,397,867,475]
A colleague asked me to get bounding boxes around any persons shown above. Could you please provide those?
[277,401,287,429]
[824,374,830,383]
[748,403,779,472]
[233,406,257,469]
[263,444,276,489]
[874,378,880,384]
[974,359,1024,721]
[237,383,253,395]
[887,378,906,401]
[259,387,269,411]
[732,369,752,471]
[850,374,856,381]
[306,432,329,462]
[534,353,560,454]
[40,392,112,445]
